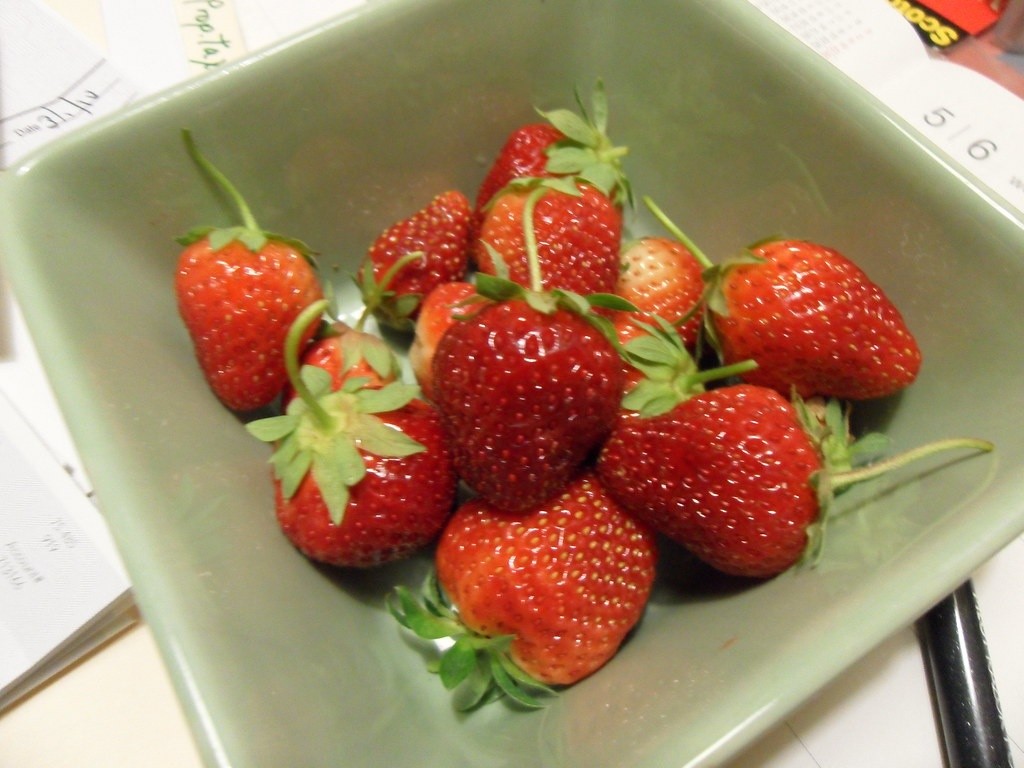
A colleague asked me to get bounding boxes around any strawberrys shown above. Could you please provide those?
[176,76,994,711]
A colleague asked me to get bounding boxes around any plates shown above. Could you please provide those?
[2,2,1024,768]
